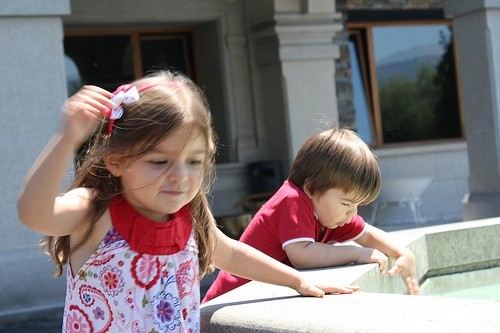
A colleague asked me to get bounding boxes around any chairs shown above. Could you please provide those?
[369,177,430,224]
[236,192,274,214]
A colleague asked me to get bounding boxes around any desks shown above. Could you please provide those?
[215,211,252,238]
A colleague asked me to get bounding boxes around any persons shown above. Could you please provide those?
[16,70,360,333]
[202,130,422,304]
